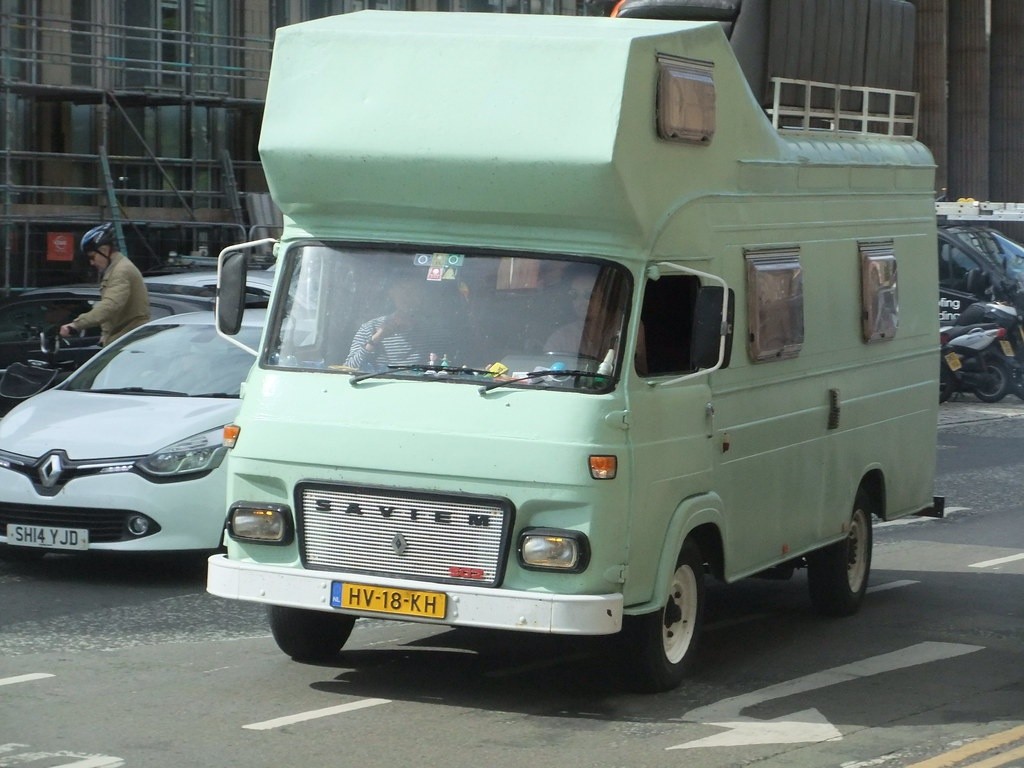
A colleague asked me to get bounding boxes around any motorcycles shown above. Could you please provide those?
[939,300,1024,406]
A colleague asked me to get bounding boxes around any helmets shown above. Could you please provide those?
[80,222,113,256]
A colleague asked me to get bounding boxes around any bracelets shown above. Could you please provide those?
[367,337,379,345]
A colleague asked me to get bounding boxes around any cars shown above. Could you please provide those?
[938,223,1024,405]
[0,246,324,571]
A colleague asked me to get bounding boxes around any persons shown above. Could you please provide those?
[345,269,457,372]
[59,222,150,348]
[545,267,647,372]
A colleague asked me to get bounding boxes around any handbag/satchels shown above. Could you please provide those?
[0,362,58,403]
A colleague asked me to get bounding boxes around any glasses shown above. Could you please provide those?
[567,288,592,300]
[88,252,97,260]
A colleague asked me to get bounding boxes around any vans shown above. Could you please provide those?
[200,8,948,699]
